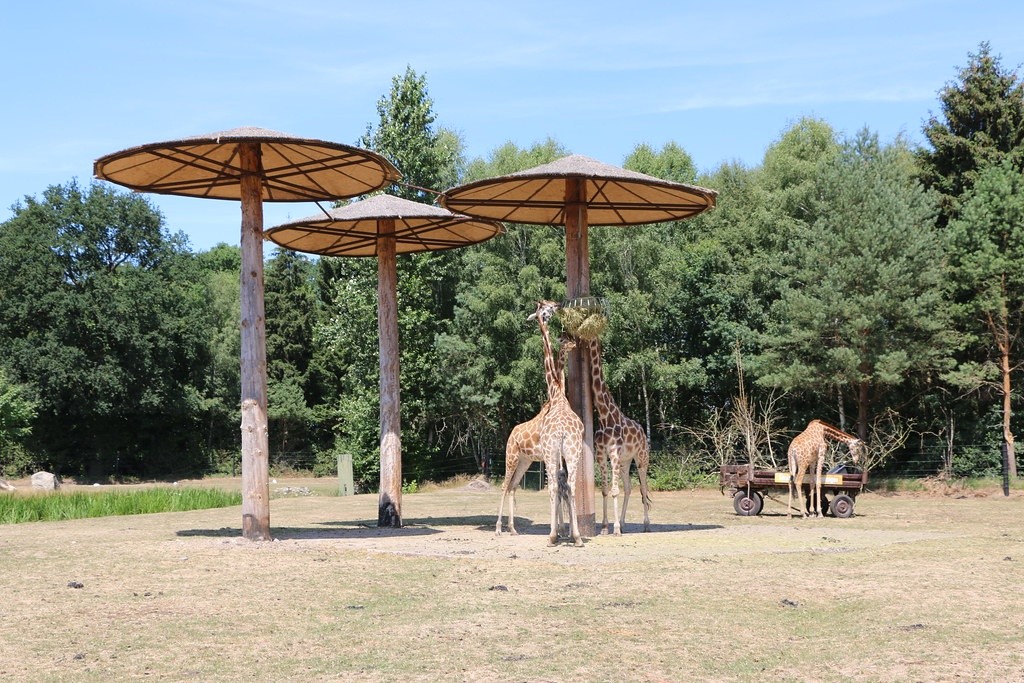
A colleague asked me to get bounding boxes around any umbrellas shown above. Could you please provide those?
[437,154,719,541]
[263,194,506,528]
[92,125,402,541]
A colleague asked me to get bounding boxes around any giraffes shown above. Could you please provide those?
[495,300,652,547]
[786,419,865,520]
